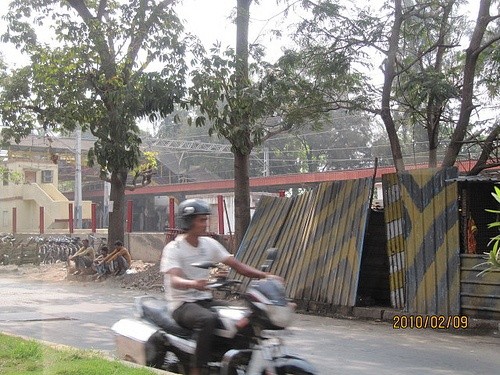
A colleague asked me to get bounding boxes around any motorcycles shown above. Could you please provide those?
[134,247,313,375]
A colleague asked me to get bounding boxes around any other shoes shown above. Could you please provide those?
[73,271,79,275]
[114,270,119,276]
[80,271,86,275]
[116,275,124,280]
[94,272,99,277]
[97,274,105,279]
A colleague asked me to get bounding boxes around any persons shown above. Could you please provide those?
[67,239,131,279]
[160,198,284,375]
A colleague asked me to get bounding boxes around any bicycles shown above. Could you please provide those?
[88,233,107,252]
[33,234,83,262]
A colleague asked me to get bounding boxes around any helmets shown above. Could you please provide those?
[176,198,213,219]
